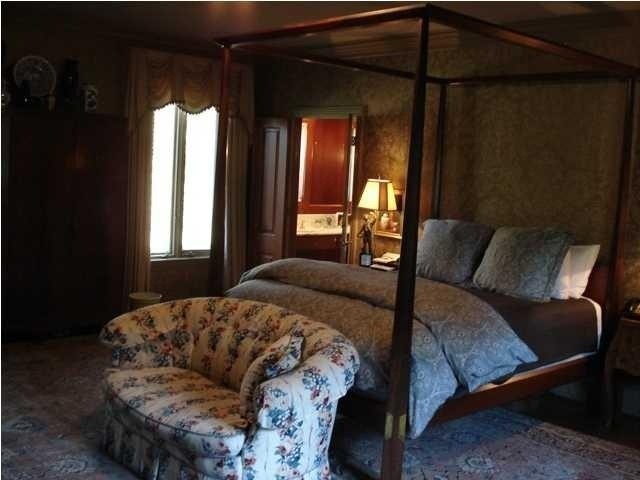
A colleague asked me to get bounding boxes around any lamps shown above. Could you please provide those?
[358,178,398,266]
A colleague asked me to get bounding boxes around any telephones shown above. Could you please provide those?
[625,298,639,318]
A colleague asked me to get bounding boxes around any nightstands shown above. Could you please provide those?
[599,315,640,435]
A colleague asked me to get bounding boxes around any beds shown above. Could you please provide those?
[208,2,640,480]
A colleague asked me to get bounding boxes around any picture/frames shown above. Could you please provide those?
[371,189,406,240]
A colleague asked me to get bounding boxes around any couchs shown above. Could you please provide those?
[98,296,360,480]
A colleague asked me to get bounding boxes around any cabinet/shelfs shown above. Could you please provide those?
[2,106,132,340]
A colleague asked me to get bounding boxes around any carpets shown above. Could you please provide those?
[2,335,640,480]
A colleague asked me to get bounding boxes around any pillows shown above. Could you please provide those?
[397,216,602,302]
[238,330,305,417]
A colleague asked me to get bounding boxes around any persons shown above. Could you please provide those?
[357,211,377,254]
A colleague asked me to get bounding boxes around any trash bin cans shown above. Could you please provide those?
[129,293,163,310]
